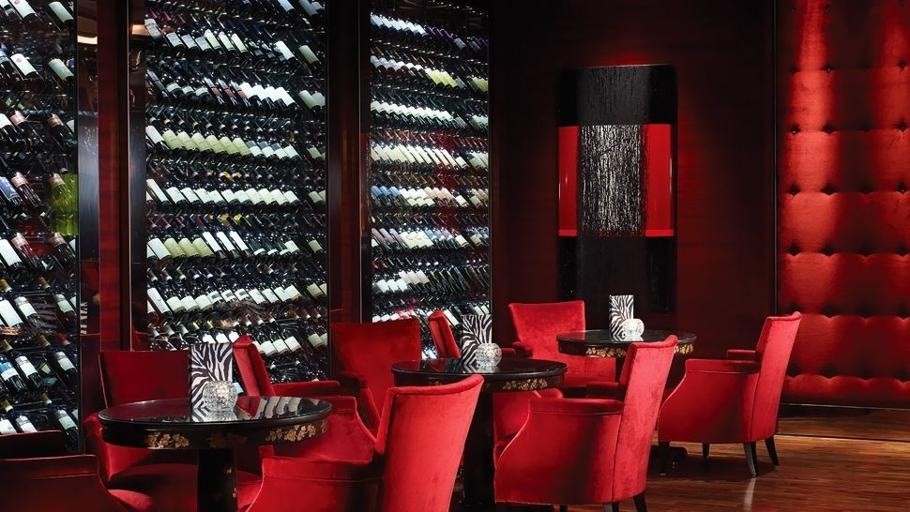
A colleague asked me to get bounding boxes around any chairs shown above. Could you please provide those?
[507,299,613,396]
[492,333,679,512]
[652,309,802,478]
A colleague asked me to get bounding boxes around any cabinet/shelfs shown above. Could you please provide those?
[0,0,82,439]
[119,0,337,385]
[336,0,494,365]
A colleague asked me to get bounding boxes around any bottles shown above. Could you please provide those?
[0,0,78,453]
[368,1,490,360]
[238,396,322,421]
[144,0,326,371]
